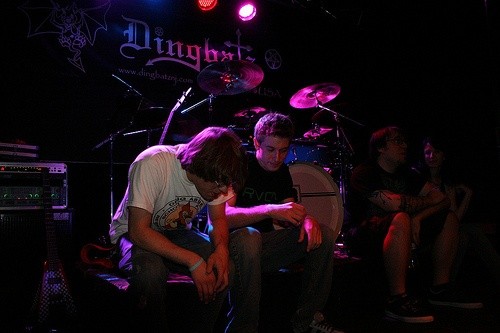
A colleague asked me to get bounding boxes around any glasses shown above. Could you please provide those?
[215,179,236,187]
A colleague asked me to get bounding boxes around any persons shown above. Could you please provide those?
[422,138,472,219]
[226,113,344,333]
[109,127,245,333]
[353,126,483,322]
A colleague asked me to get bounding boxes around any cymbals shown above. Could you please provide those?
[197,59,264,96]
[290,82,340,108]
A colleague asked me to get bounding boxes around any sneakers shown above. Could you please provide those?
[429,285,482,308]
[309,312,343,333]
[384,295,434,324]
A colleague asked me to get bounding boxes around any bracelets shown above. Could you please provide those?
[189,257,204,272]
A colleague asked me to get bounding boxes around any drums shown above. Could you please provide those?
[285,140,342,176]
[272,163,344,243]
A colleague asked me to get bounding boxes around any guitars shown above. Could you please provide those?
[27,167,80,333]
